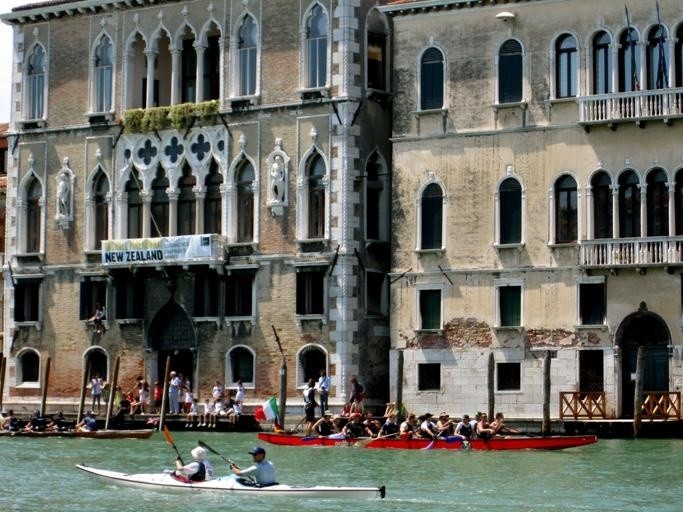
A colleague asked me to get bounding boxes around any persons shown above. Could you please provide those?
[89,373,111,414]
[114,376,163,419]
[313,411,517,440]
[168,372,244,428]
[24,409,45,432]
[230,446,275,488]
[175,446,213,484]
[93,302,106,334]
[349,378,365,413]
[318,371,330,416]
[303,379,319,437]
[56,174,69,217]
[74,410,96,432]
[47,410,67,431]
[1,410,18,432]
[270,156,284,201]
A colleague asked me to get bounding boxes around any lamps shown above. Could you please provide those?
[496,12,514,23]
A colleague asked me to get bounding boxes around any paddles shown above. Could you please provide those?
[354,433,398,448]
[163,426,185,467]
[198,440,255,482]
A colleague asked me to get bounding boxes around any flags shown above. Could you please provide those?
[256,395,280,421]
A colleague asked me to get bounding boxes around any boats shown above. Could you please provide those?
[255,429,597,451]
[0,426,157,440]
[73,463,386,499]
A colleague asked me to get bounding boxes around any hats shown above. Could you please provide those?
[249,447,265,454]
[191,446,206,460]
[324,410,482,417]
